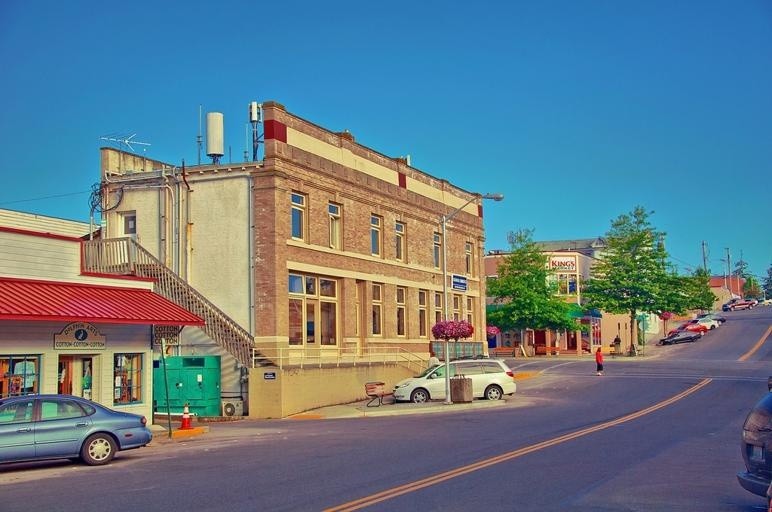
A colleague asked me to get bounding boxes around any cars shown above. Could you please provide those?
[0,394,152,466]
[393,357,517,403]
[722,296,772,312]
[656,312,727,346]
[737,375,772,512]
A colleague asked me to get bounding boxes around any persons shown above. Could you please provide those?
[596,347,603,375]
[614,334,621,355]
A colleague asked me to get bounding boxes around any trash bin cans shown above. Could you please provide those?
[631,343,638,356]
[450,373,473,404]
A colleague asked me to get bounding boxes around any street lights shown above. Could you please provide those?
[441,193,504,405]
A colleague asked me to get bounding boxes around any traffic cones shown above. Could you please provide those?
[177,403,193,430]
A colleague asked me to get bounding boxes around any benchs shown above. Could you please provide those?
[364,382,397,408]
